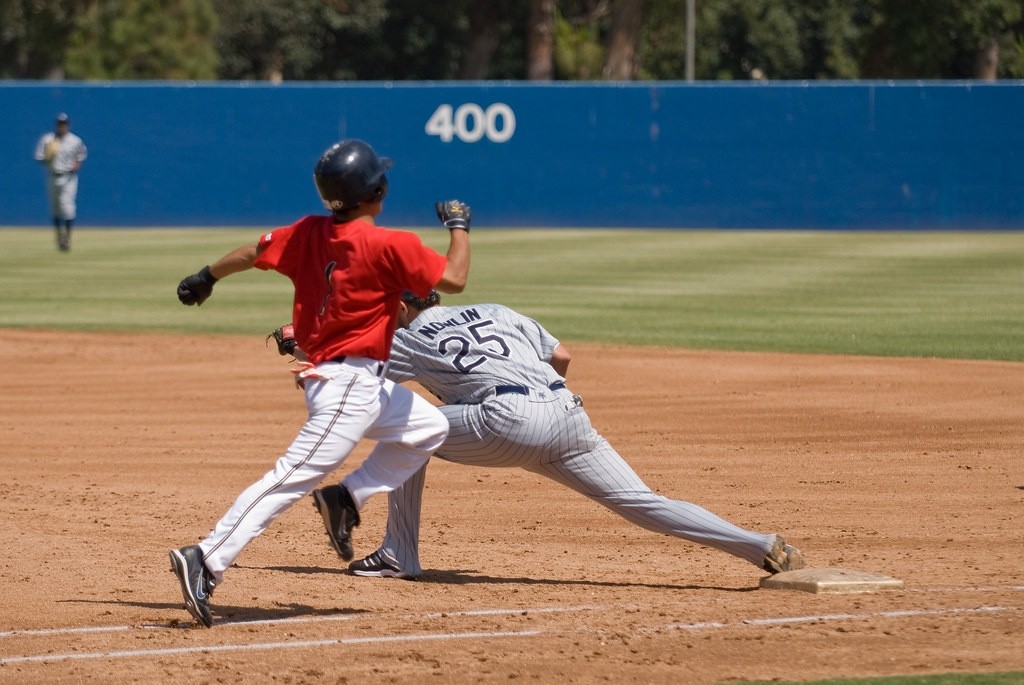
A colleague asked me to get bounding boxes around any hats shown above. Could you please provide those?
[56,112,70,123]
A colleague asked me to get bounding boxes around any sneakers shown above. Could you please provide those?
[308,484,358,560]
[169,545,216,628]
[761,533,804,575]
[349,553,406,577]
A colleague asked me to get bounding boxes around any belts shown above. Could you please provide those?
[496,383,566,396]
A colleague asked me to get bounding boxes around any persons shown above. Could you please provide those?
[33,113,87,253]
[290,286,805,581]
[168,140,471,629]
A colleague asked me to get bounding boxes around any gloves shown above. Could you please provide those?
[176,265,219,306]
[435,199,473,233]
[272,321,298,355]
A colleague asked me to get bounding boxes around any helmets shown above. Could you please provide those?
[312,138,392,211]
